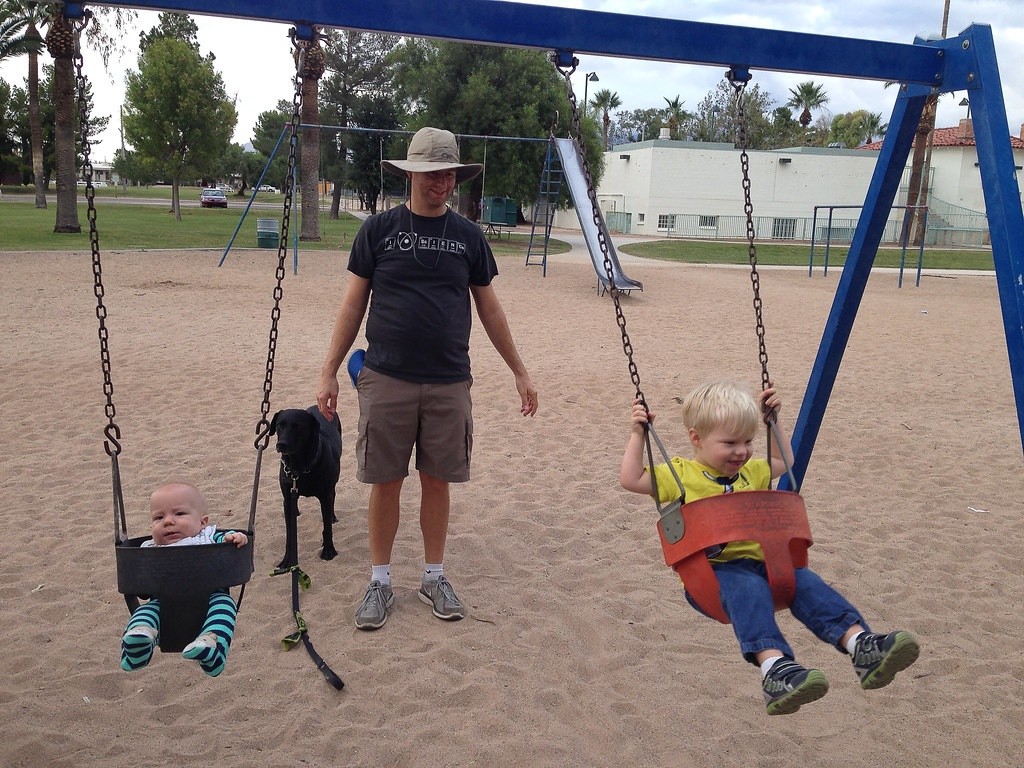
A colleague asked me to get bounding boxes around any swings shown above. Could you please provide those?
[454,134,488,234]
[60,3,316,655]
[319,128,348,249]
[379,130,411,218]
[545,50,813,624]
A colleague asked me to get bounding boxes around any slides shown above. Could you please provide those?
[551,134,645,297]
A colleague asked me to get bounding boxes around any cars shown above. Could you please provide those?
[208,183,235,193]
[198,188,228,208]
[250,184,277,192]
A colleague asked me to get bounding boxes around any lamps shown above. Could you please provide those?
[780,159,791,163]
[620,155,630,159]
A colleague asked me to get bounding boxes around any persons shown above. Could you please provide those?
[120,482,248,677]
[315,126,538,633]
[619,376,919,716]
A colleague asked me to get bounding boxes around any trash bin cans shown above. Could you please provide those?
[256,217,279,248]
[483,197,517,227]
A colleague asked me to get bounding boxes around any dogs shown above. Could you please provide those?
[267,404,343,571]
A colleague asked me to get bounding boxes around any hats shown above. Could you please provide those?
[380,127,484,269]
[347,349,366,388]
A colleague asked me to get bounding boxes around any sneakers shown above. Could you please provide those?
[417,574,464,619]
[851,630,920,690]
[353,579,394,628]
[763,657,829,715]
